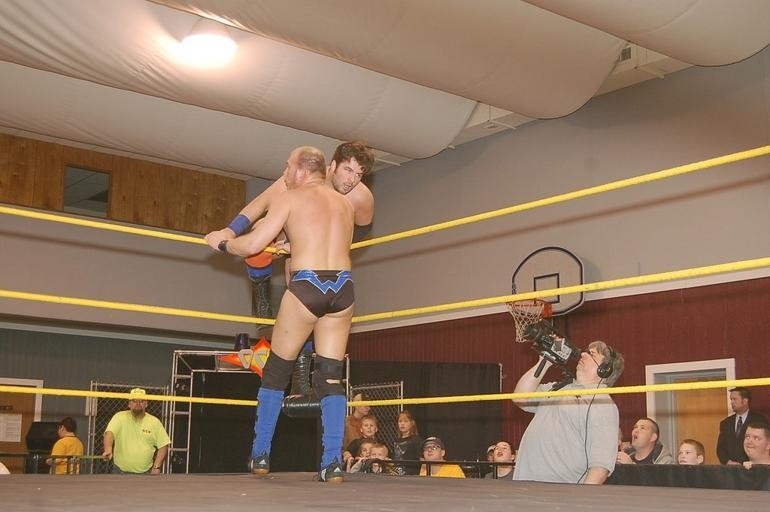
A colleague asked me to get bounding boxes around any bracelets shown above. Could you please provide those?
[228,213,252,238]
[152,465,160,469]
[218,240,228,253]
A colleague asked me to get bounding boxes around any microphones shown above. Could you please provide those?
[586,349,603,373]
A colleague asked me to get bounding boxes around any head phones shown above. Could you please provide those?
[597,345,616,378]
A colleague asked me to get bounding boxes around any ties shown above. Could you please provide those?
[736,417,742,434]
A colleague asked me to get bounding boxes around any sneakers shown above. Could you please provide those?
[318,463,343,482]
[252,277,273,329]
[250,455,270,474]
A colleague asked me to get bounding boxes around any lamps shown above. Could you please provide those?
[180,18,237,69]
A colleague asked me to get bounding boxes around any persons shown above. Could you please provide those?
[613,388,770,471]
[512,337,626,486]
[0,461,11,475]
[45,417,84,475]
[205,139,375,394]
[102,388,171,474]
[208,147,354,483]
[345,393,517,480]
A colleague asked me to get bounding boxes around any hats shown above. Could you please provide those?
[130,388,145,394]
[423,437,444,450]
